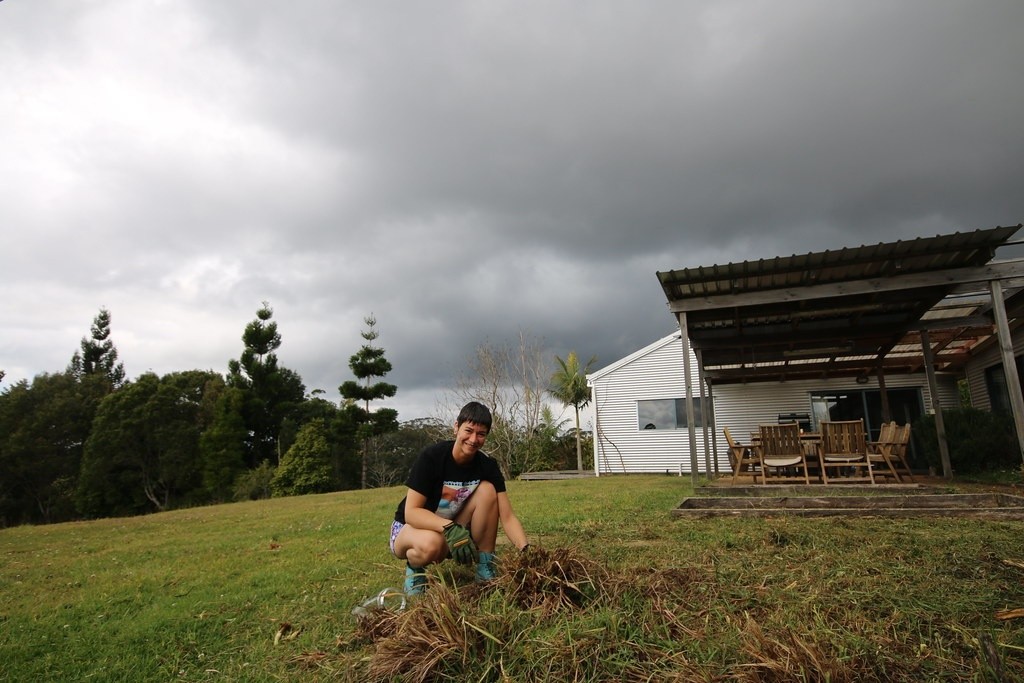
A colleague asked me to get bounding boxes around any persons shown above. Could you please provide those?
[390,401,535,600]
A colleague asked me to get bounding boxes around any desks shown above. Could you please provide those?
[749,432,867,449]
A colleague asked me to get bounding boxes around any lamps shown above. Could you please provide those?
[856,373,870,386]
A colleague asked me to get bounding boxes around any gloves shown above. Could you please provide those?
[443,522,480,567]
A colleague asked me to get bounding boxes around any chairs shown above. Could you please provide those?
[724,418,917,485]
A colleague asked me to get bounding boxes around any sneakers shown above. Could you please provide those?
[404,563,425,600]
[476,551,500,584]
